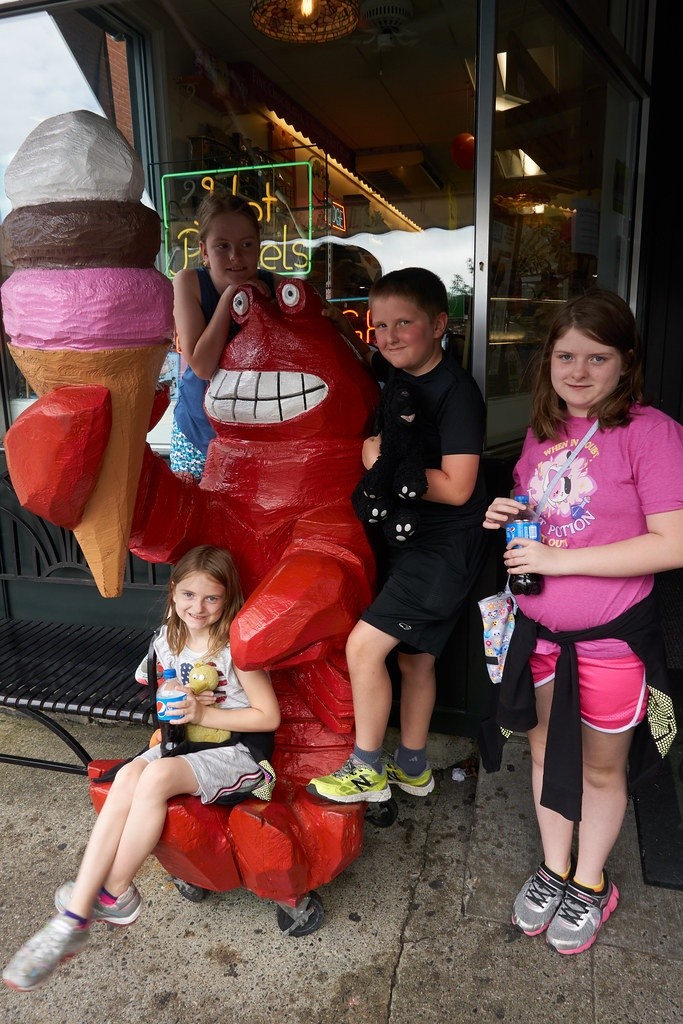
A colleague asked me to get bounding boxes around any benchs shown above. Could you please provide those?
[0,619,159,774]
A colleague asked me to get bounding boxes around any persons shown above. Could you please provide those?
[307,265,486,806]
[1,542,283,991]
[167,192,289,480]
[482,293,681,953]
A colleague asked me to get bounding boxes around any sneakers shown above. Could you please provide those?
[385,755,436,796]
[56,881,144,925]
[2,915,91,992]
[549,870,619,954]
[513,854,576,937]
[306,754,392,805]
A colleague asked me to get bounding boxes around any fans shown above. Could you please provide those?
[356,0,417,47]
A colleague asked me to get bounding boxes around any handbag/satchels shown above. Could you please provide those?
[478,593,516,684]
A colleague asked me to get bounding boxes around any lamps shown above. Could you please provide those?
[493,143,552,180]
[494,182,551,216]
[464,52,532,113]
[249,0,360,45]
[450,77,475,170]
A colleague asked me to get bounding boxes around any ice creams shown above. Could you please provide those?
[0,110,173,598]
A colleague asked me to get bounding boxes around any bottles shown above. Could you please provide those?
[505,495,543,596]
[156,669,188,758]
